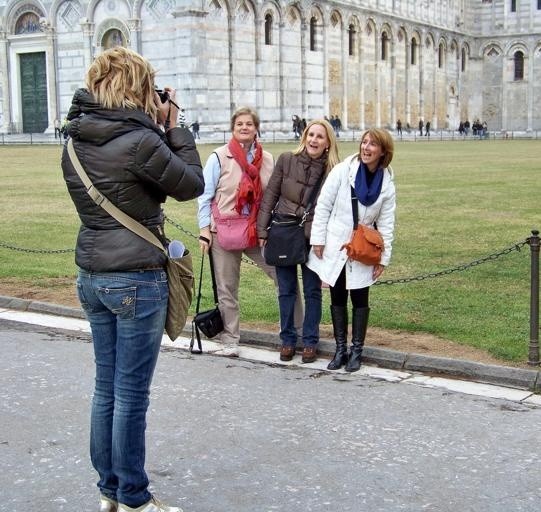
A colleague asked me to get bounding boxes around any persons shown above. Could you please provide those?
[397,118,432,137]
[291,113,344,141]
[179,109,186,128]
[255,118,344,365]
[459,117,489,139]
[59,45,206,511]
[197,107,314,357]
[189,120,201,139]
[306,127,398,374]
[60,116,70,140]
[54,118,61,139]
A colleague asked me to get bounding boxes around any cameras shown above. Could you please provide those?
[153,89,168,124]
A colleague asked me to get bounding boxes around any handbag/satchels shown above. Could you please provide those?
[190,307,225,355]
[210,198,258,251]
[262,163,328,268]
[339,184,385,267]
[162,238,196,343]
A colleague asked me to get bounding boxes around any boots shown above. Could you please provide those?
[327,305,348,371]
[345,307,370,372]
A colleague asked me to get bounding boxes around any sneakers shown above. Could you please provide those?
[280,344,296,362]
[225,342,240,357]
[301,343,319,363]
[100,493,185,512]
